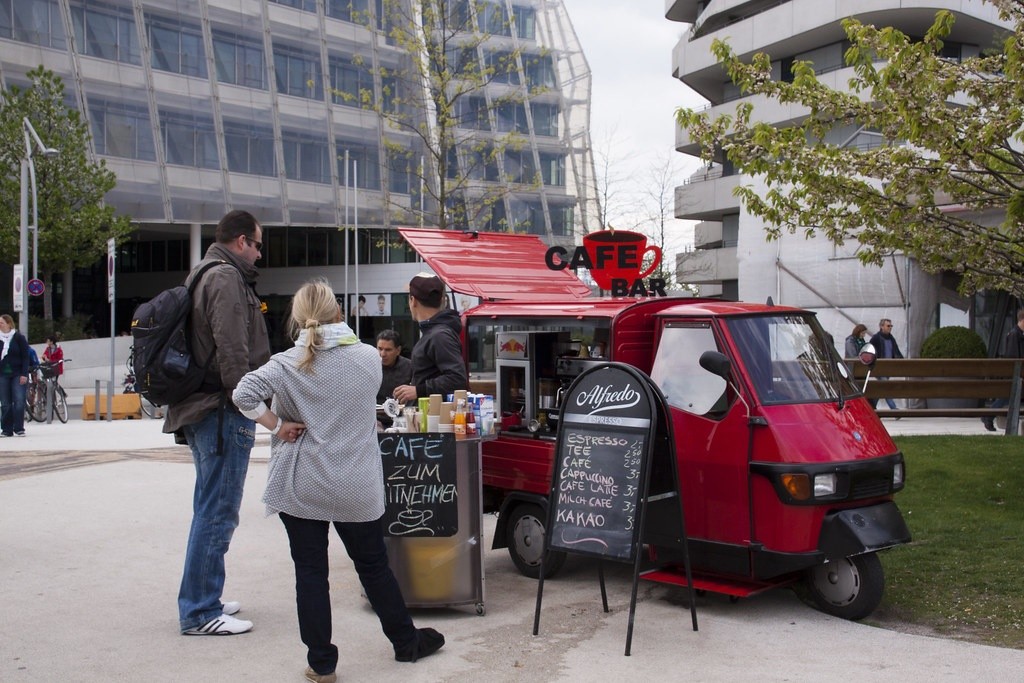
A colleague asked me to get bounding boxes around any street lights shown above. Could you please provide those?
[18,116,60,346]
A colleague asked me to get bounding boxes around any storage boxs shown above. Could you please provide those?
[83,394,142,419]
[450,393,495,436]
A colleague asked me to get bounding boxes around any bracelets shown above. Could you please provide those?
[270,416,282,436]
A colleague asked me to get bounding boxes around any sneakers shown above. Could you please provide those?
[218,601,239,614]
[186,614,252,635]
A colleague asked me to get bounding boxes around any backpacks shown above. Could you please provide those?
[131,260,244,404]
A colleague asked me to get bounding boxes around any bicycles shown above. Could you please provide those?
[138,392,164,420]
[24,358,73,423]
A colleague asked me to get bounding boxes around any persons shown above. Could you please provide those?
[233,276,445,683]
[376,329,418,432]
[351,294,368,316]
[178,209,272,634]
[372,295,390,316]
[981,308,1024,431]
[25,343,40,419]
[869,319,905,421]
[845,323,868,358]
[0,314,30,438]
[393,271,467,412]
[42,335,64,380]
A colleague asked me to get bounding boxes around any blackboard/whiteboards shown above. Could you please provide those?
[376,434,459,538]
[545,362,686,565]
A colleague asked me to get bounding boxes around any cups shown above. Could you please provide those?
[404,389,470,434]
[583,230,662,290]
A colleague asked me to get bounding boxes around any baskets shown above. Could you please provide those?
[40,361,59,379]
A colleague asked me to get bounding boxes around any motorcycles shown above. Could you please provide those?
[394,224,914,623]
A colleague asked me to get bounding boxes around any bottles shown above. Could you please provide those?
[453,399,467,435]
[465,401,477,435]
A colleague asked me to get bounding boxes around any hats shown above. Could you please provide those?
[409,272,443,303]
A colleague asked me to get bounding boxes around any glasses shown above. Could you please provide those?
[235,236,263,250]
[885,324,893,328]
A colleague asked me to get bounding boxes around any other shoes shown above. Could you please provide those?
[0,431,12,438]
[981,416,995,431]
[13,428,26,436]
[305,663,337,683]
[395,627,444,661]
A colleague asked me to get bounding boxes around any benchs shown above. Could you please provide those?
[776,357,1024,436]
